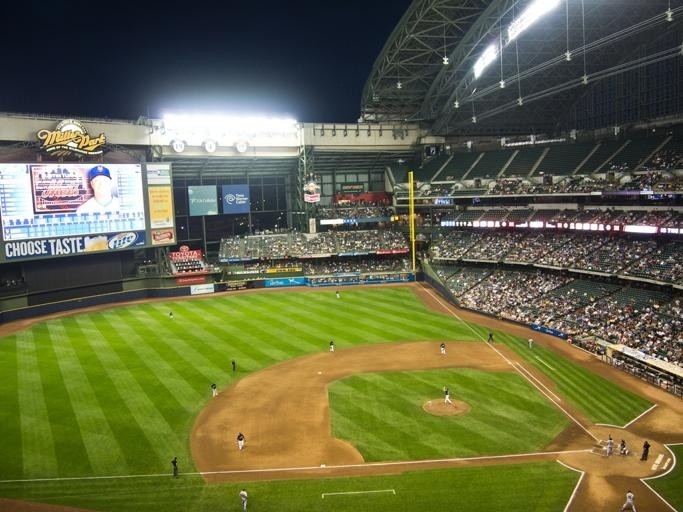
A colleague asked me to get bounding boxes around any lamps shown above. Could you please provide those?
[497,0,524,106]
[664,1,675,23]
[396,55,403,89]
[563,1,589,86]
[442,18,477,125]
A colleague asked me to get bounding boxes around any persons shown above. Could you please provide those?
[72,165,120,216]
[168,311,173,319]
[619,439,629,455]
[639,440,651,460]
[440,342,447,354]
[329,340,334,352]
[238,487,248,511]
[606,150,683,170]
[442,384,452,404]
[231,360,236,370]
[170,457,179,477]
[605,438,614,456]
[236,431,246,450]
[210,382,218,397]
[335,290,340,299]
[621,489,637,512]
[442,284,683,398]
[213,170,683,283]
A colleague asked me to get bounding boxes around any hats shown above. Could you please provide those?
[90,165,111,181]
